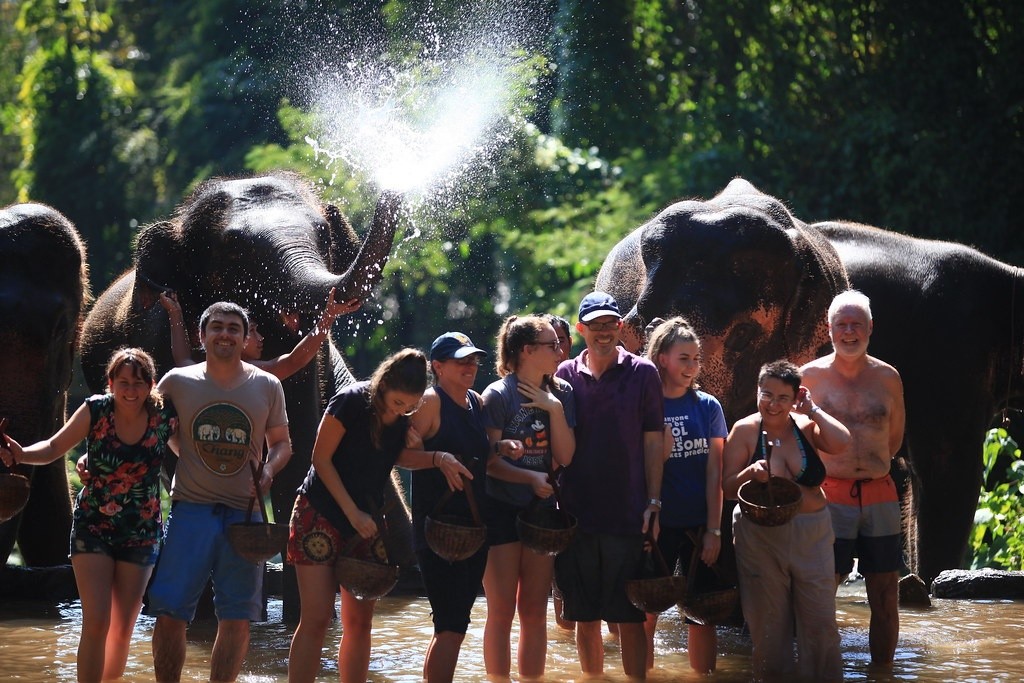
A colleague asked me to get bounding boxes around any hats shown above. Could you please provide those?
[431,332,489,359]
[579,292,622,322]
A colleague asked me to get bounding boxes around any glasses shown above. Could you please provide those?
[756,392,794,404]
[450,358,484,367]
[521,341,560,351]
[581,321,621,331]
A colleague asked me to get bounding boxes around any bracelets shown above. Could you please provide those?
[325,309,336,320]
[495,440,500,455]
[432,451,437,468]
[809,407,819,420]
[647,498,662,509]
[440,452,447,465]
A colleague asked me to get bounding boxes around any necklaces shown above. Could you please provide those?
[764,418,789,447]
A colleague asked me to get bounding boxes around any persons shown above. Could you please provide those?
[0,292,904,683]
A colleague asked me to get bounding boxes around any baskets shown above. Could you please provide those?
[1,415,29,524]
[336,490,402,600]
[737,431,803,526]
[677,538,739,625]
[624,510,684,615]
[424,455,492,562]
[516,464,581,553]
[228,460,293,561]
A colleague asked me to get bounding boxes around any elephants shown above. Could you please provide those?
[0,202,80,613]
[808,217,1024,596]
[592,174,849,639]
[74,168,415,623]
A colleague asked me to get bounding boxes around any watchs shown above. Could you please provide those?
[705,527,721,537]
